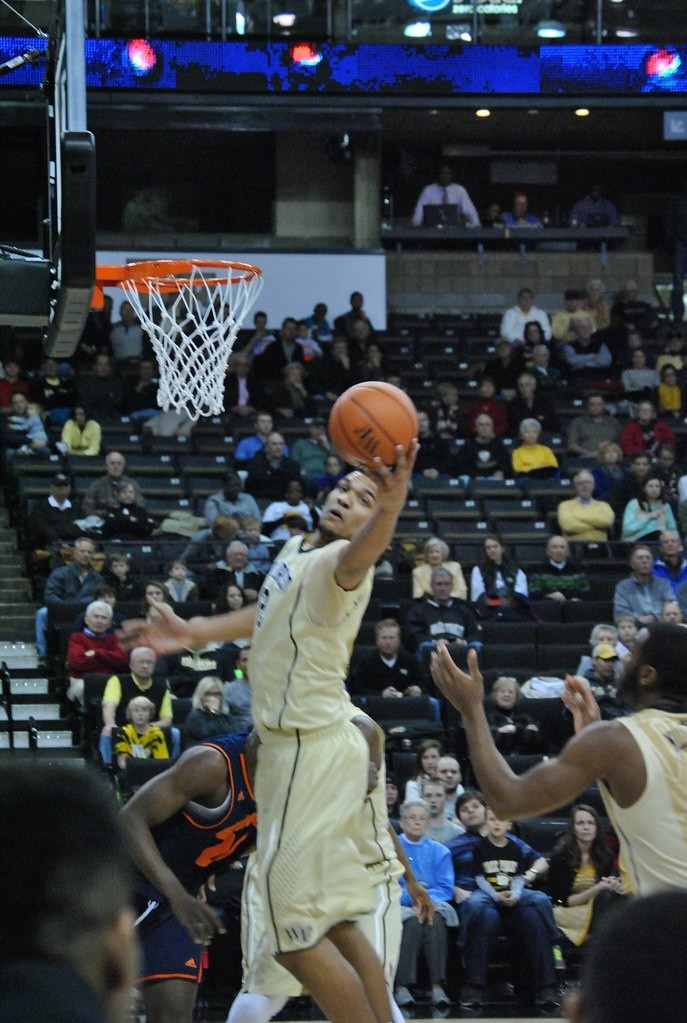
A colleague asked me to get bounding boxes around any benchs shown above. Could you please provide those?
[0,311,687,985]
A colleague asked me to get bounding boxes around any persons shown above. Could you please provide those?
[0,154,687,1023]
[115,434,420,1023]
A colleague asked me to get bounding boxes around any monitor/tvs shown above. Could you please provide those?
[423,204,463,229]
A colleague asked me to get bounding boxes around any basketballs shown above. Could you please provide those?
[329,381,419,469]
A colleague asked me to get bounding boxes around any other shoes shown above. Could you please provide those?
[552,944,566,969]
[534,987,561,1006]
[459,990,483,1007]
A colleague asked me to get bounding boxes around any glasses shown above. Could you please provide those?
[204,691,222,696]
[498,676,517,682]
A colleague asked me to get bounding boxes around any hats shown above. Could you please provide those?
[386,772,397,783]
[592,644,619,660]
[307,417,326,426]
[52,473,69,483]
[494,337,510,347]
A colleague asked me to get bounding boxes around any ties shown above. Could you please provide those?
[441,187,447,204]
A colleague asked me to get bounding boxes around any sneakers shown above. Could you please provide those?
[432,987,450,1006]
[394,987,416,1006]
[432,1007,450,1019]
[400,1007,415,1019]
